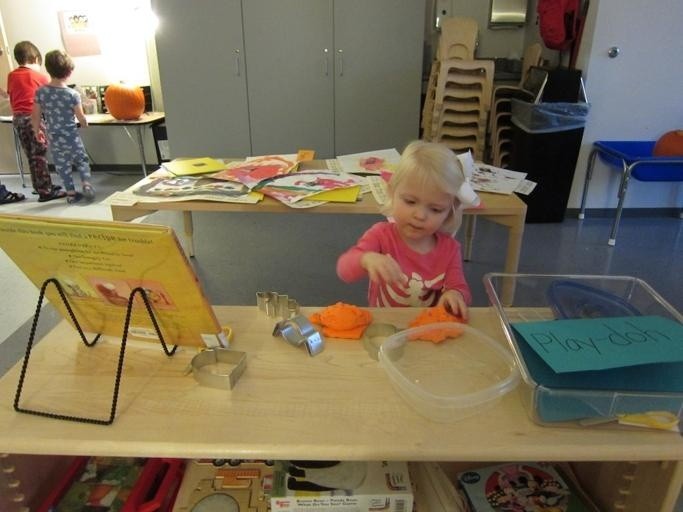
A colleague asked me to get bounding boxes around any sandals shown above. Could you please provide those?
[83,185,95,198]
[0,192,25,204]
[66,192,82,203]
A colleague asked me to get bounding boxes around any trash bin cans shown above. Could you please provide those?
[506,65,590,224]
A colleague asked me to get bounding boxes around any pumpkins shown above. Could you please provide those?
[105,82,145,121]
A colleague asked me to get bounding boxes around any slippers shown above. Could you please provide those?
[32,185,61,194]
[38,191,67,202]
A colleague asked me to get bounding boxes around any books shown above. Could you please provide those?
[453,461,595,511]
[1,211,222,350]
[270,459,414,512]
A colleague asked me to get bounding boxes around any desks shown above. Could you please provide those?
[110,158,527,307]
[0,306,683,511]
[0,112,164,188]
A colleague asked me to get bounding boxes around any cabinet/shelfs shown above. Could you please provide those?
[241,0,426,159]
[153,0,252,159]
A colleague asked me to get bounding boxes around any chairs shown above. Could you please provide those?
[421,16,550,169]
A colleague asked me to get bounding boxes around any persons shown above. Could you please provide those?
[6,41,66,201]
[30,50,95,204]
[335,138,473,321]
[0,182,27,206]
